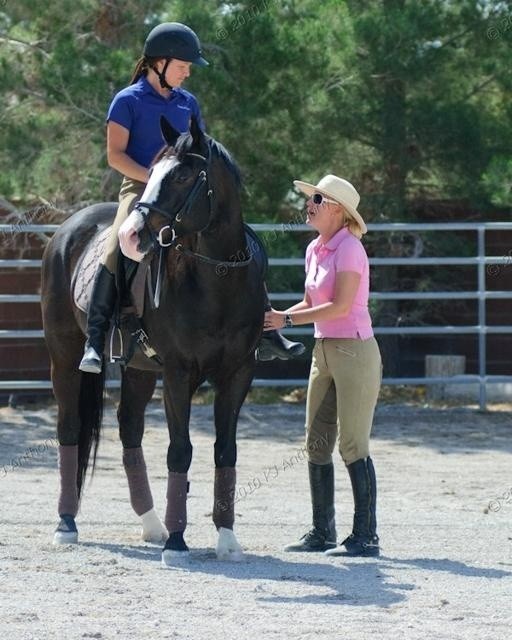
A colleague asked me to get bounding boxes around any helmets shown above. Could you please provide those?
[143,23,211,68]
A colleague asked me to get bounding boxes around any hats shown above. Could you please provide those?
[294,175,368,235]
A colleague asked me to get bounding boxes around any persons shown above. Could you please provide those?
[80,20,308,373]
[262,170,383,558]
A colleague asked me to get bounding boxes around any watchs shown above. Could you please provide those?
[281,313,293,328]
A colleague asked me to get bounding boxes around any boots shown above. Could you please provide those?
[285,461,337,552]
[325,456,379,556]
[79,263,115,374]
[255,282,306,361]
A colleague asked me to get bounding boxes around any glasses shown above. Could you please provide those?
[311,192,339,206]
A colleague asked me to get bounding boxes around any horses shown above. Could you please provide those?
[40,113,269,567]
